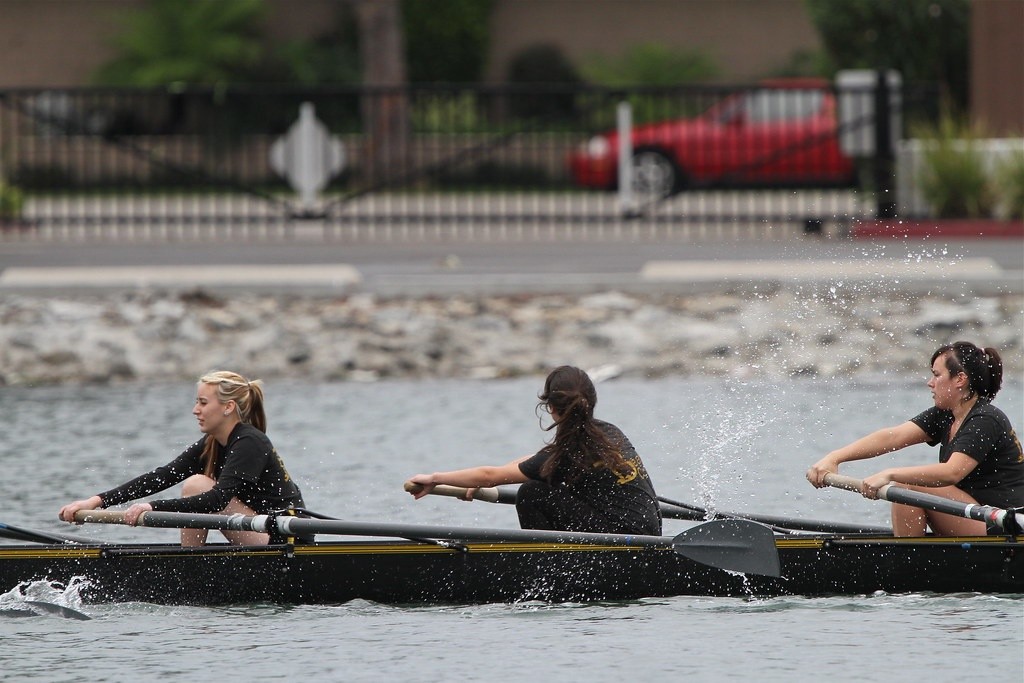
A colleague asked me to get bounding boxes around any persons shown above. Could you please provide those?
[806,341,1024,537]
[58,370,315,545]
[410,365,663,537]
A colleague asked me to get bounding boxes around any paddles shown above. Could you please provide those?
[0,522,109,545]
[805,467,1024,534]
[58,508,782,579]
[403,480,894,534]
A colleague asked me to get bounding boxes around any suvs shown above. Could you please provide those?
[568,73,880,205]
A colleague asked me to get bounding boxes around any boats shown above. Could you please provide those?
[0,503,1024,610]
